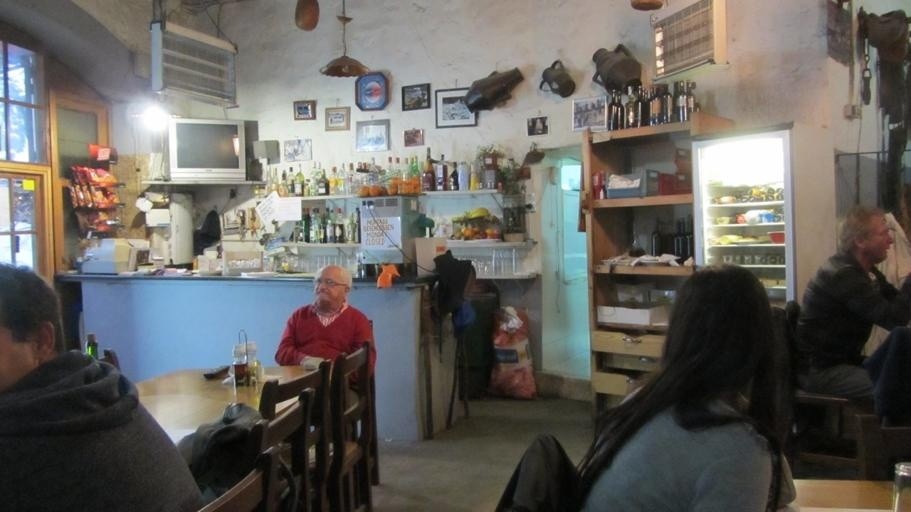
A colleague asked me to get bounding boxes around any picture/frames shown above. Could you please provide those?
[324,105,352,134]
[433,88,479,128]
[572,95,608,132]
[402,128,426,147]
[354,71,388,111]
[292,100,316,122]
[354,120,392,155]
[400,85,431,112]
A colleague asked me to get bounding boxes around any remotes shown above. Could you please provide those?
[204,365,230,379]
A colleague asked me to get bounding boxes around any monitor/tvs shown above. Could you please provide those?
[168,117,246,181]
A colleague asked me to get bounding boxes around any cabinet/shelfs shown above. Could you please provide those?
[70,180,128,234]
[581,111,736,423]
[420,188,537,283]
[253,194,365,248]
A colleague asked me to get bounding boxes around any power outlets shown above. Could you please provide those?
[846,100,864,117]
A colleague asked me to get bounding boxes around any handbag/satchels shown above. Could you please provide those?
[504,434,585,512]
[180,401,263,500]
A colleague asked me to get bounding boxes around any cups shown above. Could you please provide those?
[289,253,355,272]
[257,366,265,382]
[705,182,786,302]
[151,256,165,277]
[452,247,530,277]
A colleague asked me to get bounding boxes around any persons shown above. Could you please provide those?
[572,264,793,511]
[793,206,911,416]
[275,261,379,388]
[0,260,207,511]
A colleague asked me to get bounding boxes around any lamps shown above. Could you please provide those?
[647,0,730,87]
[320,1,368,77]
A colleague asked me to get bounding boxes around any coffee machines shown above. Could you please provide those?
[144,191,197,274]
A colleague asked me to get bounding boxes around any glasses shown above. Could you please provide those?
[314,279,347,286]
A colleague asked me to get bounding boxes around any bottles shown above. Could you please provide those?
[893,460,911,512]
[247,159,355,195]
[421,146,481,192]
[84,331,99,358]
[232,343,248,387]
[245,341,259,388]
[592,44,643,94]
[293,201,374,244]
[355,153,421,196]
[540,59,575,98]
[465,67,525,114]
[628,214,694,266]
[608,78,701,130]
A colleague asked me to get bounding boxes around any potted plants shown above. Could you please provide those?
[476,142,539,241]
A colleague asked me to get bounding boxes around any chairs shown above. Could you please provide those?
[784,300,877,477]
[198,319,380,512]
[496,432,577,512]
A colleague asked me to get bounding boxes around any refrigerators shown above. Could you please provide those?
[689,119,843,327]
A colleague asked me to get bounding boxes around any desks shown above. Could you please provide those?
[788,479,910,512]
[132,364,318,447]
